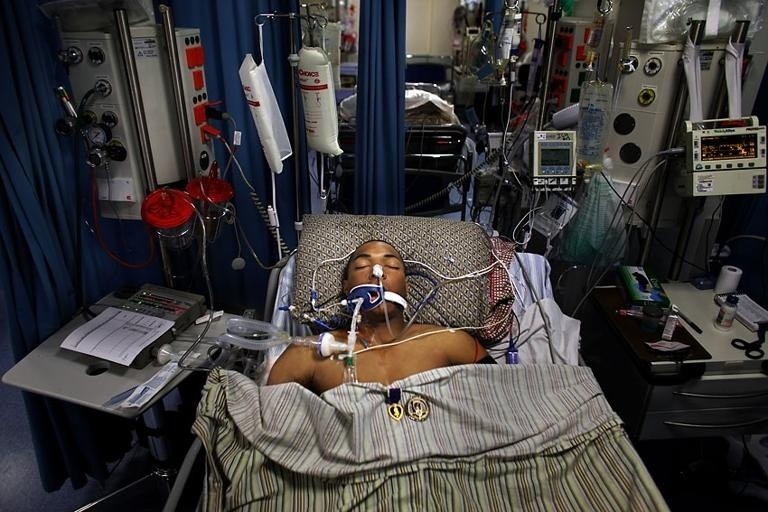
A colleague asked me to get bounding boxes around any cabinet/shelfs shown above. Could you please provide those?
[714,294,739,332]
[641,305,663,333]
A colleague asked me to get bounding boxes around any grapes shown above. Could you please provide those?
[590,281,768,441]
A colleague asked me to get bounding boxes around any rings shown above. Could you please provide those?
[678,310,703,334]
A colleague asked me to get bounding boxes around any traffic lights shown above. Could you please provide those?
[291,215,489,333]
[340,92,457,126]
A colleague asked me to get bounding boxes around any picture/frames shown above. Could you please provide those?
[2,292,249,511]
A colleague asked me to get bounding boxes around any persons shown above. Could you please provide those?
[267,239,499,392]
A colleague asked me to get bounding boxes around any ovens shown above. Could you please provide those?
[319,83,475,222]
[166,250,670,512]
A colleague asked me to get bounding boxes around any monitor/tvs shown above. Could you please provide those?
[716,291,768,332]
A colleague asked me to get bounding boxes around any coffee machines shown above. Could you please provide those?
[731,338,765,359]
